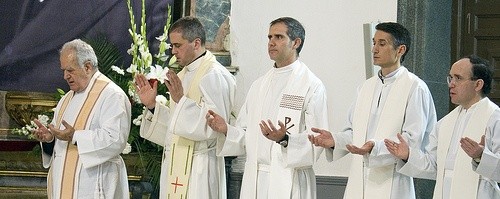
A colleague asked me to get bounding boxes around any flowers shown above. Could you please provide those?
[11,0,183,186]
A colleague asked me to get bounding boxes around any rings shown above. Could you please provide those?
[266,133,269,136]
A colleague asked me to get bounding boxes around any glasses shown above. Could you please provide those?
[446,75,482,84]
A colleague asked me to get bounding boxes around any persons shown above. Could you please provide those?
[385,55,500,199]
[134,16,235,199]
[206,16,328,199]
[31,38,130,199]
[309,21,437,199]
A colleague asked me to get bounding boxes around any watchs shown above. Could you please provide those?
[277,134,288,147]
